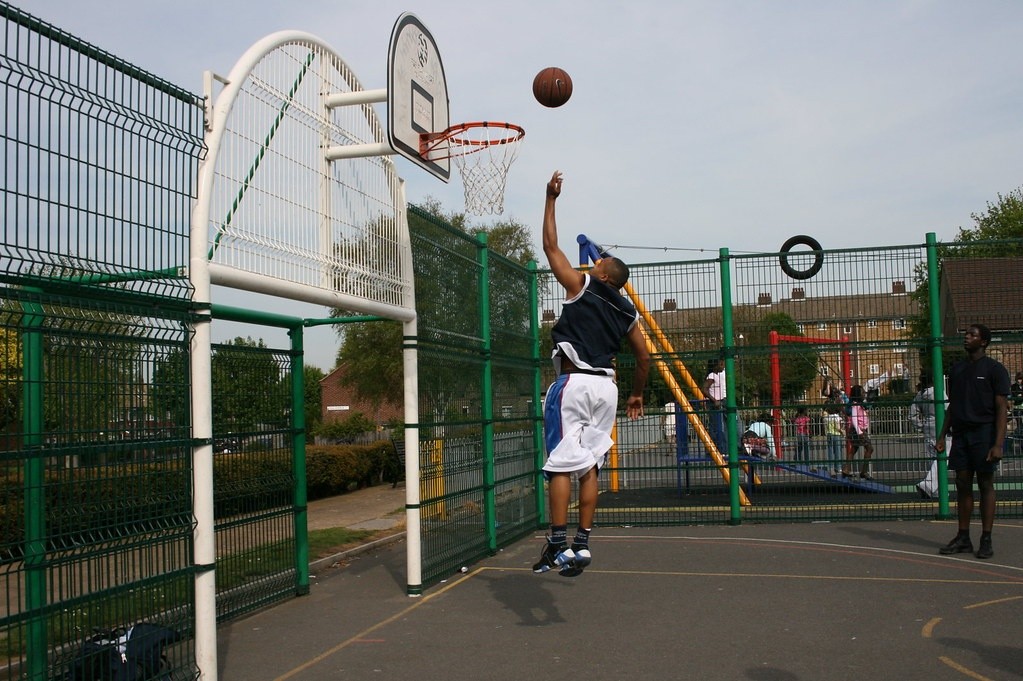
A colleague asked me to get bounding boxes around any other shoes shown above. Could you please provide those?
[916,483,932,498]
[837,469,842,474]
[827,470,836,477]
[842,471,857,477]
[860,471,874,480]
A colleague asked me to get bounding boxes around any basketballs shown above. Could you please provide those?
[532,66,573,108]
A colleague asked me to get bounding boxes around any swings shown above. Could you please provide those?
[786,342,856,417]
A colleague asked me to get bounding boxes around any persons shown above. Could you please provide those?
[788,406,812,466]
[742,413,778,461]
[1011,373,1023,436]
[820,378,873,482]
[935,324,1011,558]
[702,357,741,459]
[533,169,650,577]
[911,370,953,499]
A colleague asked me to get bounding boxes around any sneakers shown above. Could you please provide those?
[939,535,973,554]
[976,541,993,557]
[558,535,592,577]
[533,533,576,573]
